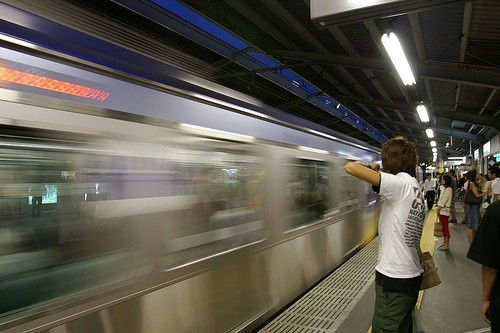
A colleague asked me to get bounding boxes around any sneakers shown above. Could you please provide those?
[437,245,449,251]
[448,219,457,223]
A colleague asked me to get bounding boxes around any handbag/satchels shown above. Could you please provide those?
[433,213,443,237]
[418,247,442,290]
[463,182,483,204]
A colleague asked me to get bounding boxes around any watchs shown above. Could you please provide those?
[367,164,372,169]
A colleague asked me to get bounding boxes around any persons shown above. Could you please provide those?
[436,175,452,251]
[467,169,492,246]
[0,123,379,223]
[424,173,436,211]
[488,166,500,203]
[424,161,500,224]
[343,136,429,330]
[447,169,457,223]
[467,199,500,332]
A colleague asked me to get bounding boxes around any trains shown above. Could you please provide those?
[0,1,424,333]
